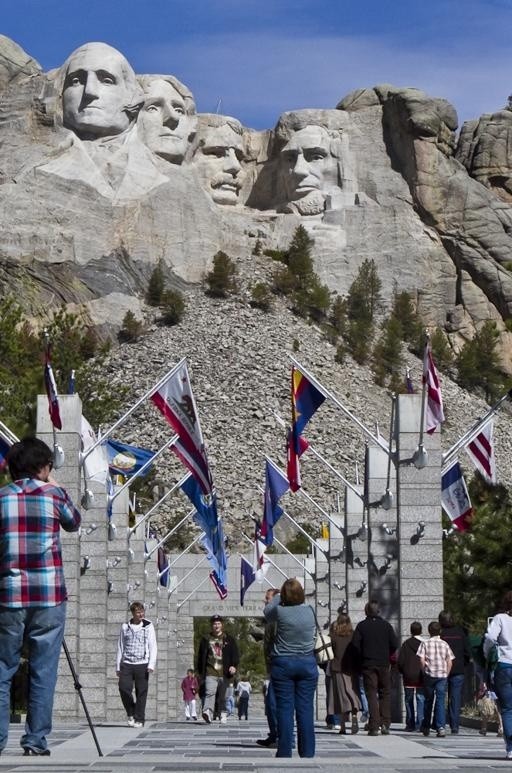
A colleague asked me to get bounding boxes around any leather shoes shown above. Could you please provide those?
[22,747,51,757]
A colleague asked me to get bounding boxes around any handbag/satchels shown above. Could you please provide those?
[476,657,498,723]
[309,604,335,664]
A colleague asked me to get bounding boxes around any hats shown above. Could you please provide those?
[210,614,223,622]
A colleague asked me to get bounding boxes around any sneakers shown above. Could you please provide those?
[381,724,389,735]
[367,729,380,736]
[202,711,212,724]
[436,727,446,737]
[127,716,136,726]
[219,712,228,724]
[133,721,144,728]
[478,729,486,736]
[256,737,279,748]
[419,724,430,737]
[496,729,503,737]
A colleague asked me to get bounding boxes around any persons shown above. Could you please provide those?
[38,42,168,203]
[262,680,270,704]
[131,73,196,161]
[476,668,503,737]
[184,114,247,204]
[400,621,425,732]
[274,109,358,215]
[483,591,512,759]
[431,610,465,734]
[114,603,158,726]
[416,621,456,737]
[235,675,252,720]
[269,579,319,758]
[198,615,239,723]
[326,613,362,734]
[353,600,399,736]
[358,676,369,722]
[255,588,297,749]
[225,683,235,716]
[181,669,199,721]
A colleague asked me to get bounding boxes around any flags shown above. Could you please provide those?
[199,522,227,567]
[210,572,230,599]
[285,426,308,493]
[0,438,81,756]
[101,435,156,477]
[423,341,446,435]
[264,491,284,545]
[254,522,270,583]
[464,418,497,480]
[240,558,255,606]
[192,512,219,553]
[441,462,474,533]
[152,363,209,477]
[169,434,213,494]
[265,460,289,524]
[44,341,62,431]
[291,365,326,453]
[181,474,219,526]
